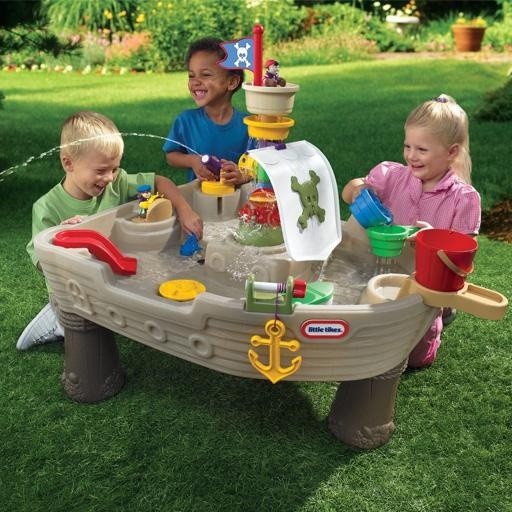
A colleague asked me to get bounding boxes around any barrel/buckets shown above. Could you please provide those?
[414,228,478,292]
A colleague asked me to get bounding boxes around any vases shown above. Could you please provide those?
[387,15,417,38]
[451,24,488,53]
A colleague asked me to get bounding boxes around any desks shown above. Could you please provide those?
[32,174,509,449]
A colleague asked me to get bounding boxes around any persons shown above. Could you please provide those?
[135,184,152,218]
[158,37,259,190]
[16,109,207,353]
[341,91,483,371]
[262,59,287,87]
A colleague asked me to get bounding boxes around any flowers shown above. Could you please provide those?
[453,15,490,25]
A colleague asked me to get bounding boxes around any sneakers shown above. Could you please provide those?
[441,307,456,326]
[16,303,64,352]
